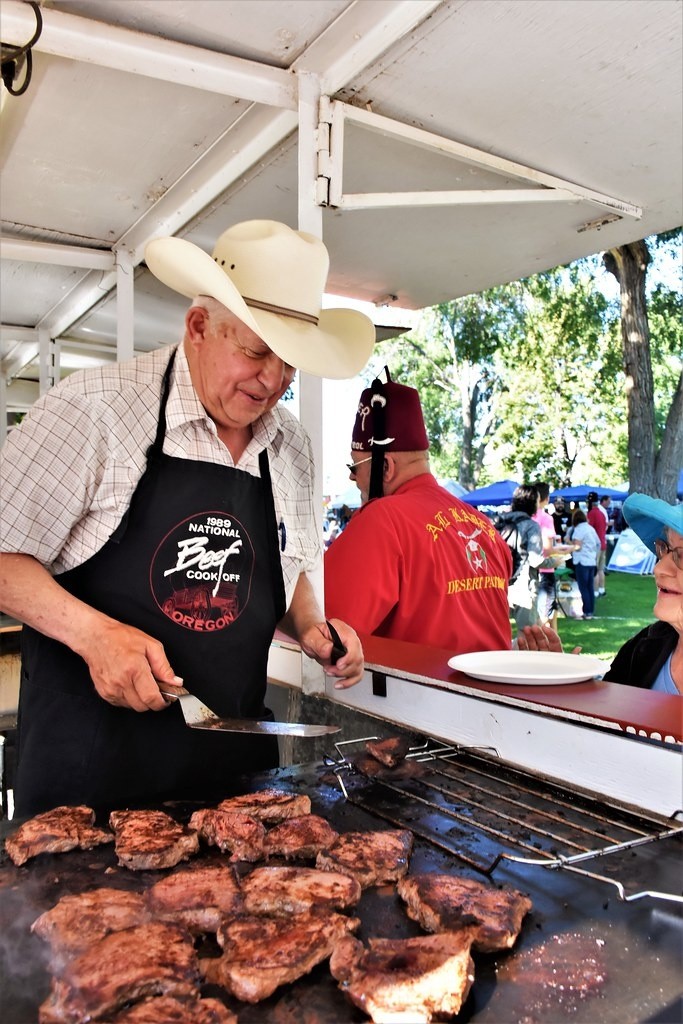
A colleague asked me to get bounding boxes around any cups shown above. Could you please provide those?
[561,524,567,531]
[610,519,614,524]
[556,535,562,545]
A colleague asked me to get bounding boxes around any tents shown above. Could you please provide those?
[327,478,627,512]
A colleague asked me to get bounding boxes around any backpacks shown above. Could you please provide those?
[494,516,530,581]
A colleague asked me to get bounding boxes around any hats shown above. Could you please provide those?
[622,492,683,558]
[352,381,428,451]
[144,220,376,381]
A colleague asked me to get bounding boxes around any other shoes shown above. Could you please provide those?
[575,614,592,620]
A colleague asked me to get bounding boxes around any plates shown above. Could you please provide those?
[553,545,574,550]
[448,651,611,685]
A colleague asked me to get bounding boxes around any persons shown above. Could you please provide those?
[478,481,629,632]
[323,383,513,653]
[0,217,376,822]
[516,491,683,696]
[321,504,357,552]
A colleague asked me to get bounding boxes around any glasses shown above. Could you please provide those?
[653,539,683,569]
[345,456,397,475]
